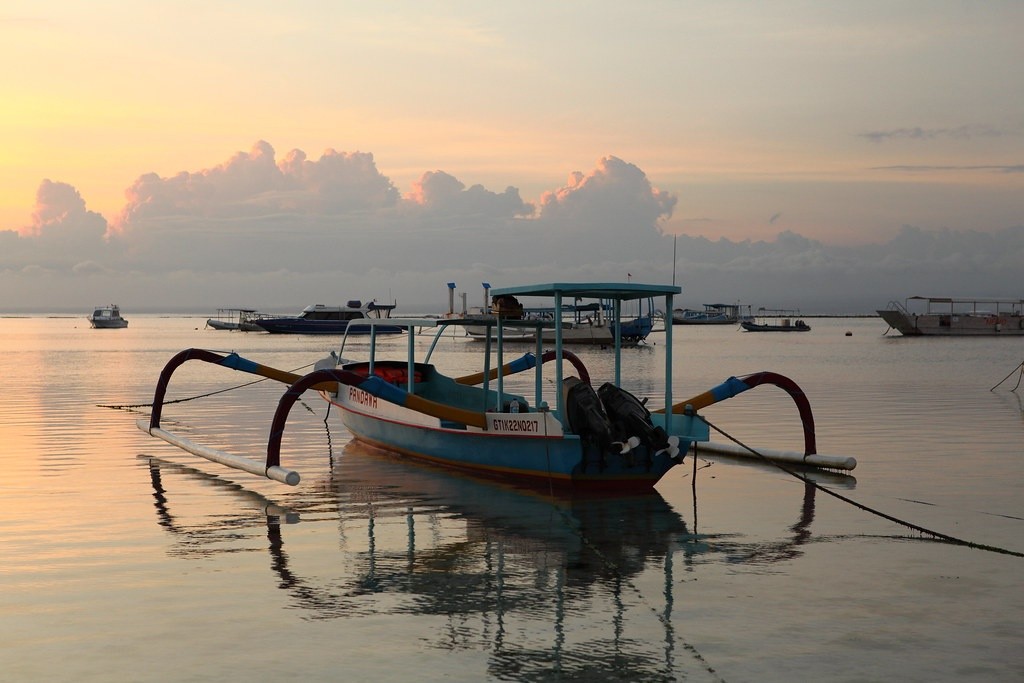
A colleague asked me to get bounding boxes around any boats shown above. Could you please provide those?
[741,307,812,331]
[207,308,258,330]
[876,293,1024,336]
[86,303,129,328]
[845,331,853,336]
[315,279,712,498]
[461,297,655,340]
[251,299,402,334]
[673,302,753,323]
[238,312,273,332]
